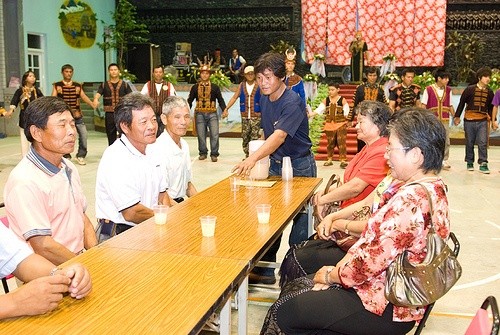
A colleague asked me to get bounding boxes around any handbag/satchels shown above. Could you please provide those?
[384,182,462,307]
[314,174,343,230]
[332,206,372,252]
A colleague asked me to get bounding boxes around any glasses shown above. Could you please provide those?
[386,146,412,155]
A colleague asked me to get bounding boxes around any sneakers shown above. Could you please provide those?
[74,156,86,164]
[249,271,276,284]
[442,159,451,168]
[479,162,490,173]
[466,161,474,170]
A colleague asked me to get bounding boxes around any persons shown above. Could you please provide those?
[153,94,197,207]
[492,88,500,131]
[187,65,228,162]
[93,92,172,245]
[454,68,498,174]
[140,65,176,138]
[0,105,10,119]
[3,95,98,288]
[7,71,44,159]
[231,53,317,284]
[345,67,389,153]
[310,100,394,240]
[349,31,370,85]
[389,68,422,115]
[259,106,450,335]
[0,219,93,320]
[221,66,262,161]
[212,49,246,82]
[277,167,406,290]
[421,70,458,168]
[309,82,353,169]
[51,64,98,165]
[94,63,133,146]
[282,54,311,116]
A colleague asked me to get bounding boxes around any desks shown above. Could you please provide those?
[0,171,323,335]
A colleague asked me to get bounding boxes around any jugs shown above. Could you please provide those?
[249,140,269,179]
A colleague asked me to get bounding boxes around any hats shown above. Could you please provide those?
[244,66,254,73]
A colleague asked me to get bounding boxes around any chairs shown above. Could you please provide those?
[370,233,500,335]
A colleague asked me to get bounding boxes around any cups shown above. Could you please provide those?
[200,216,217,237]
[230,177,240,191]
[282,156,293,180]
[245,176,255,189]
[255,204,271,223]
[153,205,169,225]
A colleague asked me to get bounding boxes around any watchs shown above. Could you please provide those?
[325,267,336,285]
[344,220,353,235]
[50,267,63,275]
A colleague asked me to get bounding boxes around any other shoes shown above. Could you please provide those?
[199,154,208,159]
[323,161,333,165]
[340,162,348,168]
[211,154,218,161]
